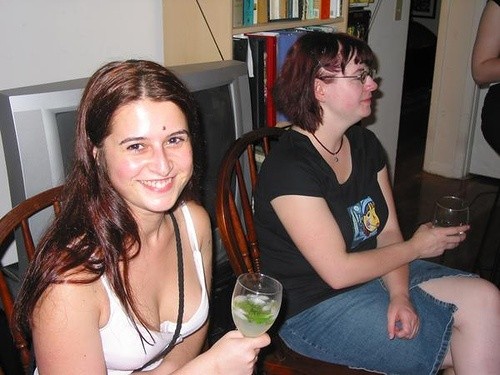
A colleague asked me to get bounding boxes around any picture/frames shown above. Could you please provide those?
[410,0,437,19]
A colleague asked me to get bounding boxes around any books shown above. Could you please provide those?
[232,0,344,128]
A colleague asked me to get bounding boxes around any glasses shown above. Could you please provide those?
[317,70,372,84]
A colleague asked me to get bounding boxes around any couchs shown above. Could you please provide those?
[400,23,437,140]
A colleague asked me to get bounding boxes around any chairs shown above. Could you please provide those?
[0,126,300,375]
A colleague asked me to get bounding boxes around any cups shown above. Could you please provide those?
[432,196,470,229]
[231,271,282,339]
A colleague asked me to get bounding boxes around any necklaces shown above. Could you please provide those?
[312,132,344,162]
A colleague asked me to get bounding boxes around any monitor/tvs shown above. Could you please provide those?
[0,59,253,272]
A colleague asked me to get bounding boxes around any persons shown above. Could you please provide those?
[252,32,500,375]
[471,0,500,157]
[14,60,271,375]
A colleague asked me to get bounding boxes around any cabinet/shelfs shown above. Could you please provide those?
[163,0,349,161]
